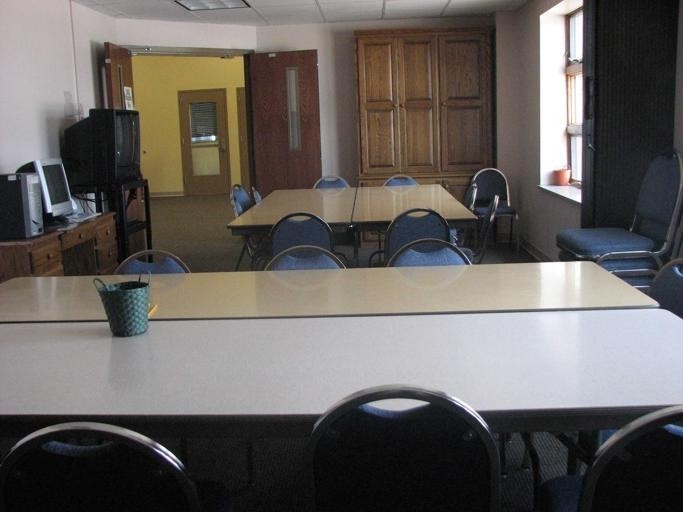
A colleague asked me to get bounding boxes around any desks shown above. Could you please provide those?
[0,259,666,321]
[0,307,683,471]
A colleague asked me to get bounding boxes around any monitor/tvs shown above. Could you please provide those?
[63,108,140,185]
[16,157,76,218]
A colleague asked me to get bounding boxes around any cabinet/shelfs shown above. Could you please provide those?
[436,27,494,167]
[351,28,439,169]
[1,211,124,275]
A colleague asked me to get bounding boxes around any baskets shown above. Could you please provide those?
[94,269,152,336]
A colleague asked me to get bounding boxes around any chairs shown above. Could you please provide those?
[211,170,503,271]
[302,377,507,512]
[386,237,518,479]
[553,143,683,315]
[466,167,519,249]
[529,398,683,509]
[0,416,201,511]
[104,247,193,275]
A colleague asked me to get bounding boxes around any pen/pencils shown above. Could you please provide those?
[147,305,157,316]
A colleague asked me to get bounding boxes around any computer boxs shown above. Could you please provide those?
[1,172,45,240]
